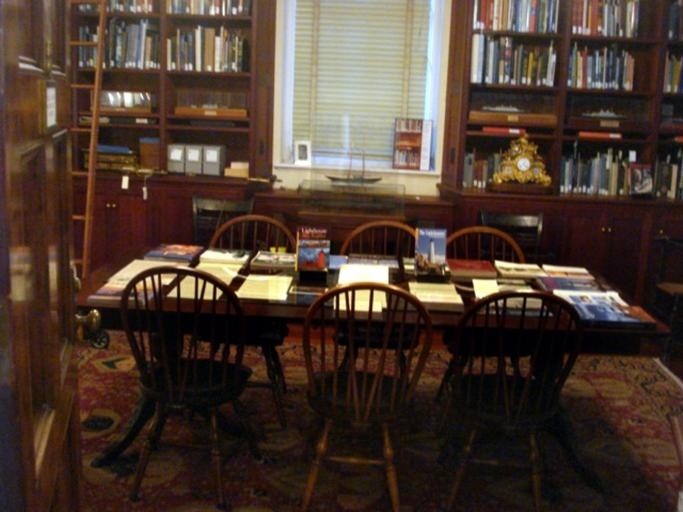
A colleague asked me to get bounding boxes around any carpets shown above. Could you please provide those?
[73,321,683,512]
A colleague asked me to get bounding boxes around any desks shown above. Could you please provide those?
[256,183,454,259]
[86,238,673,511]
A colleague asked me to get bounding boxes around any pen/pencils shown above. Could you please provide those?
[429,240,436,264]
[320,249,324,270]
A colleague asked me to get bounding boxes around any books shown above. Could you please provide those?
[89,239,657,331]
[464,0,683,204]
[77,0,252,76]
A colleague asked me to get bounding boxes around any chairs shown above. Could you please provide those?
[436,225,556,410]
[476,208,544,260]
[90,264,257,509]
[440,289,585,509]
[332,217,425,434]
[297,281,435,509]
[648,236,681,361]
[198,215,300,427]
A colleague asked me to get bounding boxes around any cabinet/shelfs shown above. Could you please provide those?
[645,198,682,318]
[438,188,557,263]
[561,198,652,311]
[149,179,268,250]
[68,173,153,307]
[68,0,274,179]
[440,0,681,198]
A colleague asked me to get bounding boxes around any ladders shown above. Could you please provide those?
[63,0,107,291]
